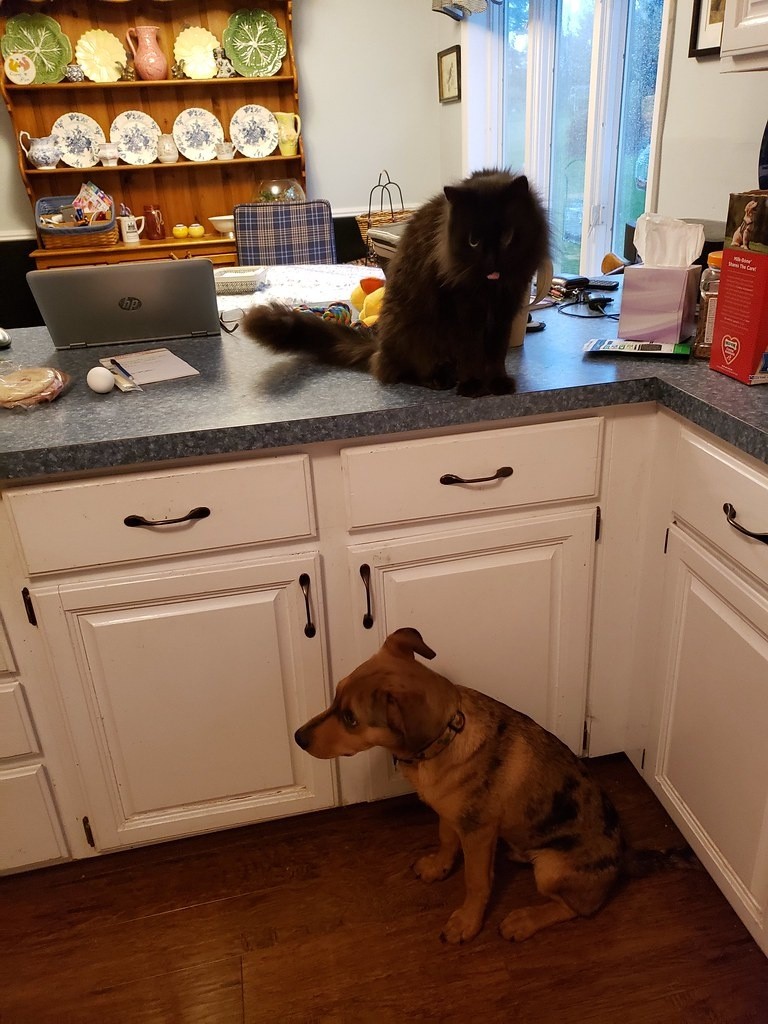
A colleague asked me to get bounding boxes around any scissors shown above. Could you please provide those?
[120,203,131,216]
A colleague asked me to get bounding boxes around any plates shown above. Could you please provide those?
[75,29,128,82]
[4,54,36,85]
[222,7,287,78]
[172,108,224,162]
[110,110,163,165]
[0,12,72,84]
[173,26,220,79]
[51,112,106,168]
[229,104,278,158]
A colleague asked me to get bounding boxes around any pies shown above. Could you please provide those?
[0,366,65,408]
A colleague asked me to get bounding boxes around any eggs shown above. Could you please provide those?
[87,366,115,394]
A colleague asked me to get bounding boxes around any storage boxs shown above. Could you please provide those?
[724,188,768,244]
[709,242,768,387]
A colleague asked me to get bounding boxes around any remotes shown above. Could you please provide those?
[588,280,619,289]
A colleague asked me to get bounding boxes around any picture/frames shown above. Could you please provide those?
[687,0,725,59]
[437,44,462,103]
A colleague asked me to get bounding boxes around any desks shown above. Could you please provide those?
[28,236,239,271]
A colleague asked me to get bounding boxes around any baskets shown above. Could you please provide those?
[35,195,119,248]
[355,209,417,249]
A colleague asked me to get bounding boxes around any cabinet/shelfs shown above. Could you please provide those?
[0,0,307,233]
[0,398,768,956]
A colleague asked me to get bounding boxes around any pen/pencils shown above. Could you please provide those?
[74,209,90,225]
[110,358,134,380]
[534,283,564,297]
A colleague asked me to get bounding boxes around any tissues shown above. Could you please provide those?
[617,211,706,344]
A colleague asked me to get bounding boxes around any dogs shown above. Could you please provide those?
[293,627,710,945]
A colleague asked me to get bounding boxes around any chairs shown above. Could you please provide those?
[232,199,338,265]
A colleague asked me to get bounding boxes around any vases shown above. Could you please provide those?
[156,131,180,164]
[97,138,122,165]
[126,24,169,80]
[16,128,61,170]
[273,111,302,156]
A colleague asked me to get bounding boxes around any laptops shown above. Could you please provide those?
[26,258,222,351]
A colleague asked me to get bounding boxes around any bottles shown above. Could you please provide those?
[694,251,723,358]
[172,224,205,239]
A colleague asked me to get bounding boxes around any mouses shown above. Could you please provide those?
[0,327,11,349]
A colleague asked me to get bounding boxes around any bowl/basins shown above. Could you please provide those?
[208,215,234,232]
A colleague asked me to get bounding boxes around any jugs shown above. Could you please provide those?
[144,204,165,240]
[19,130,65,170]
[272,111,301,156]
[115,203,145,242]
[126,26,168,80]
[61,65,84,82]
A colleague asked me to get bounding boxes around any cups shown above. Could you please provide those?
[155,134,179,164]
[92,141,121,166]
[215,141,241,160]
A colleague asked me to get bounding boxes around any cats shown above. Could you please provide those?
[242,163,563,399]
